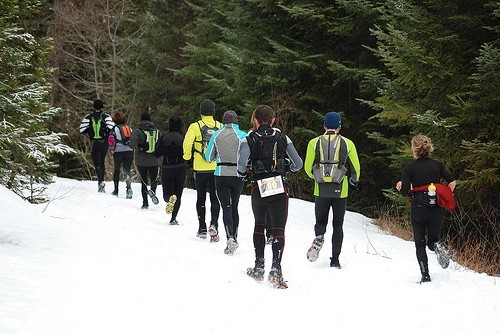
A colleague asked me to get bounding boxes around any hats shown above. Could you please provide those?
[200,99,214,116]
[141,112,149,120]
[324,112,340,129]
[92,99,105,108]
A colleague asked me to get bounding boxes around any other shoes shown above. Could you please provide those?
[420,275,430,282]
[434,243,449,268]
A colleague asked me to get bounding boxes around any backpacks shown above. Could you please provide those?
[141,128,158,153]
[198,120,220,161]
[90,113,105,140]
[249,131,287,176]
[116,124,131,143]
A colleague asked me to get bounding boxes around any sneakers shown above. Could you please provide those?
[170,219,178,226]
[165,195,177,214]
[266,238,273,244]
[112,190,119,195]
[247,267,264,281]
[148,190,158,204]
[98,183,106,192]
[330,259,341,269]
[142,206,147,209]
[197,229,207,238]
[209,226,220,242]
[224,237,238,255]
[306,234,324,263]
[267,271,287,288]
[127,189,133,199]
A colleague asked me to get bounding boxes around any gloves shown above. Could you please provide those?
[350,177,358,186]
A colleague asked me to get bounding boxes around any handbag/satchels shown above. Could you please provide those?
[413,189,437,207]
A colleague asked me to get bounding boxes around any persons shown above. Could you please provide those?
[107,112,134,199]
[396,134,457,284]
[236,106,303,288]
[204,110,247,255]
[153,116,186,225]
[303,112,361,270]
[183,101,225,243]
[130,113,161,210]
[79,99,115,193]
